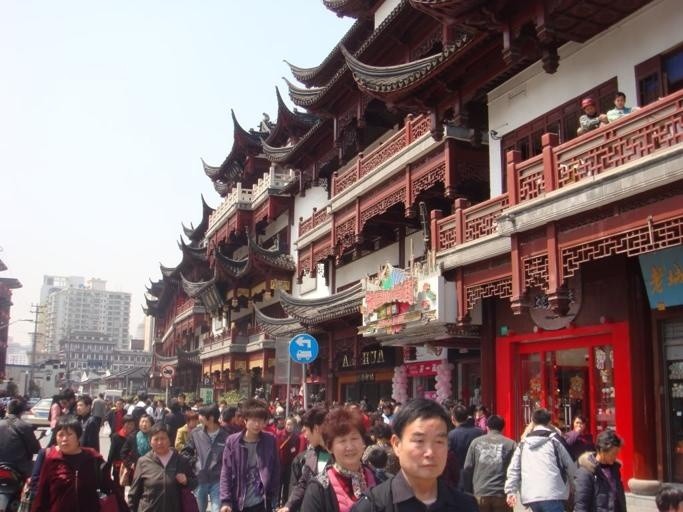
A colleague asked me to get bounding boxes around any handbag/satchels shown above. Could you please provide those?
[180,487,199,512]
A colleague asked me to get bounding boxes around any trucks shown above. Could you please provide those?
[101,389,124,411]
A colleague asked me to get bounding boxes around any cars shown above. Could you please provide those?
[0,396,53,429]
[74,393,88,399]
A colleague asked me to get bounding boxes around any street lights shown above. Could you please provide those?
[0,319,32,329]
[22,370,28,398]
[59,385,62,394]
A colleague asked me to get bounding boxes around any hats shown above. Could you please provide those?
[52,388,75,399]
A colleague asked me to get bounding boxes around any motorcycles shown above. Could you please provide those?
[0,428,51,511]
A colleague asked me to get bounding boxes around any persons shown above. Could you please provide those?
[576,98,607,137]
[0,388,628,512]
[605,91,640,124]
[655,482,683,512]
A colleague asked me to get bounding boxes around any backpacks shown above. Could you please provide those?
[132,404,147,426]
